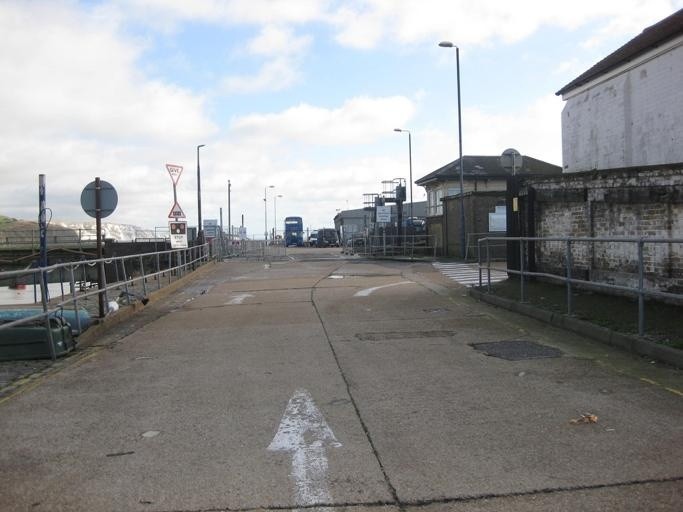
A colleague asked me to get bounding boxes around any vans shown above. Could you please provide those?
[310,227,340,248]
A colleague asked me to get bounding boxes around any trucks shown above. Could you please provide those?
[285,216,303,247]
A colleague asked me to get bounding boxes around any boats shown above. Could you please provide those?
[0,305,90,361]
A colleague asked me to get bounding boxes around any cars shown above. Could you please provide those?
[408,216,426,232]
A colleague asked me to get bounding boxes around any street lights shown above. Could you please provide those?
[264,185,283,246]
[394,128,413,222]
[439,40,467,259]
[197,144,206,245]
